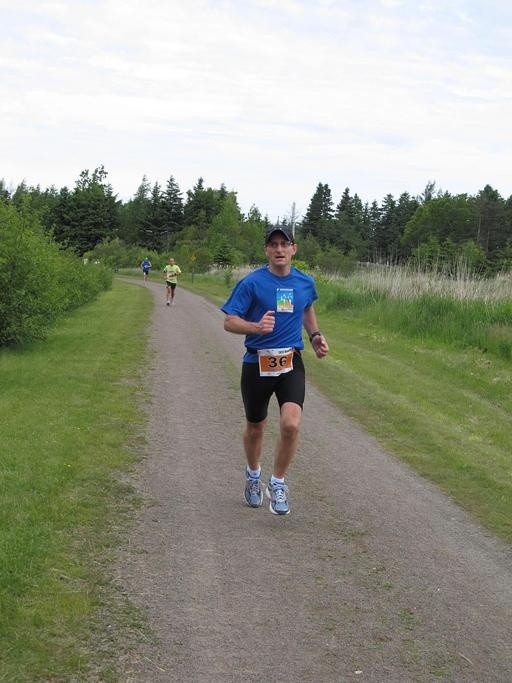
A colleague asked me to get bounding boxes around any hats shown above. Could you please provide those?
[265,225,294,245]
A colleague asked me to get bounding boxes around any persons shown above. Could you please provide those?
[162,257,182,307]
[140,255,153,283]
[221,227,329,515]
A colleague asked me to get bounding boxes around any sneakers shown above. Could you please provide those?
[243,464,263,508]
[266,478,290,515]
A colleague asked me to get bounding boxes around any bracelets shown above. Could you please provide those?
[309,331,322,343]
